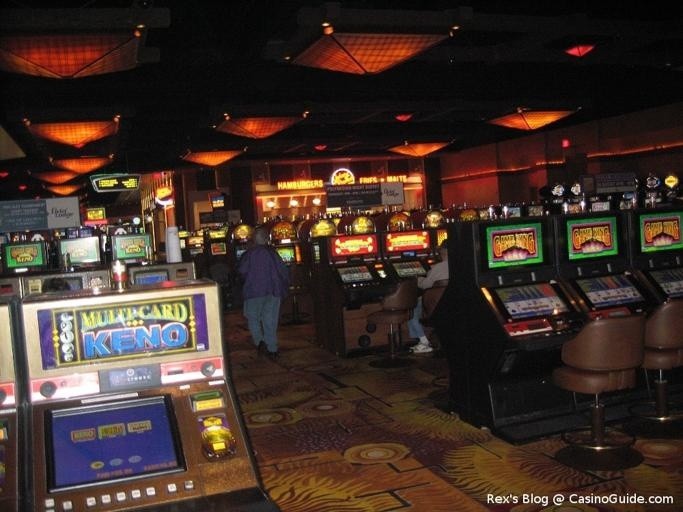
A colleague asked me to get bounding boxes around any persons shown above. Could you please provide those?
[401,239,449,354]
[235,228,291,362]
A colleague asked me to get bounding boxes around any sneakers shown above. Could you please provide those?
[409,341,434,354]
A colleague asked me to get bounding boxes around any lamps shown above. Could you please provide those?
[0,2,583,197]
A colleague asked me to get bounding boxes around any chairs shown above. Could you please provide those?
[622,297,683,441]
[367,277,419,370]
[282,260,312,328]
[543,314,647,472]
[418,279,451,362]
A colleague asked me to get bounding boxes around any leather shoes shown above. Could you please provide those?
[269,352,280,361]
[258,341,265,359]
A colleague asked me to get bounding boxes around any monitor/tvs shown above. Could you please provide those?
[189,191,683,323]
[0,226,187,495]
